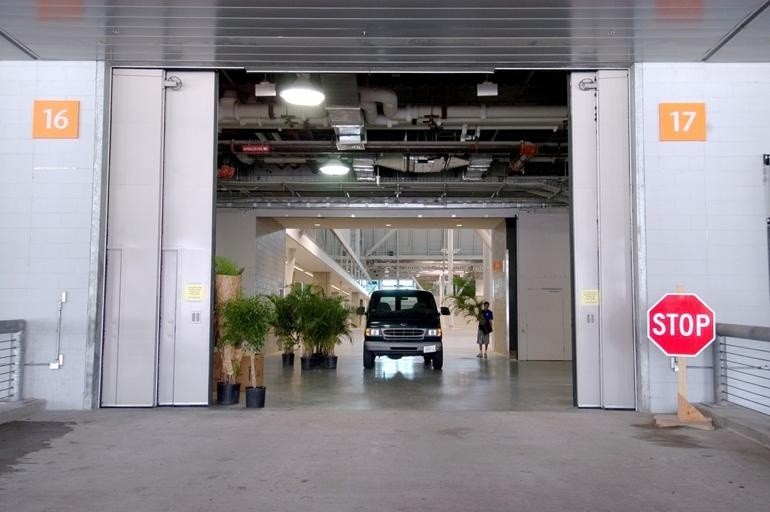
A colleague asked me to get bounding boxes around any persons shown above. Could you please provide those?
[475,301,494,358]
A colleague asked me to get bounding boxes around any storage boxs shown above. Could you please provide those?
[213,345,265,393]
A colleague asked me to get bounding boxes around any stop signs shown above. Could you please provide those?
[645,293,717,358]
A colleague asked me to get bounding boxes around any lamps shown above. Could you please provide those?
[278,71,327,108]
[319,155,349,175]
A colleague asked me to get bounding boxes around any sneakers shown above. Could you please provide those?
[477,353,482,357]
[484,354,487,357]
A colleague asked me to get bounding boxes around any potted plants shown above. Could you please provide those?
[276,281,353,372]
[213,252,246,404]
[227,297,279,408]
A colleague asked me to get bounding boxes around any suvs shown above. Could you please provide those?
[356,288,452,372]
